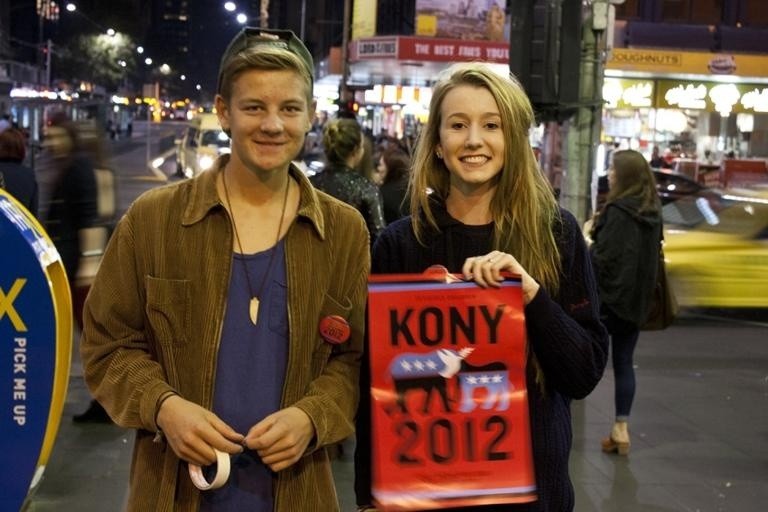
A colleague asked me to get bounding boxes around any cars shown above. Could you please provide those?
[591,160,768,245]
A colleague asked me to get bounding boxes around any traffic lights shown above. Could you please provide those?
[342,101,359,112]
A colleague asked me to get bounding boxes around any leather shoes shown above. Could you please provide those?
[73,399,112,422]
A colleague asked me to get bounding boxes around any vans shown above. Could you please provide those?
[174,109,232,181]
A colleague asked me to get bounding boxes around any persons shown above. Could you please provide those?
[348,58,609,512]
[80,26,371,511]
[652,124,750,188]
[0,94,137,325]
[291,118,421,248]
[584,146,675,455]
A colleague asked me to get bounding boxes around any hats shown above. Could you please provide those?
[216,26,318,140]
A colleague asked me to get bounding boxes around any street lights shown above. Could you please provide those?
[42,3,253,98]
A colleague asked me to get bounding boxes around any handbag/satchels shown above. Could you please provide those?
[645,280,680,331]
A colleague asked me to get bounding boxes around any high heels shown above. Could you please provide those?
[601,433,630,456]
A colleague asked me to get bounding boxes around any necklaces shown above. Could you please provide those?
[222,167,291,328]
